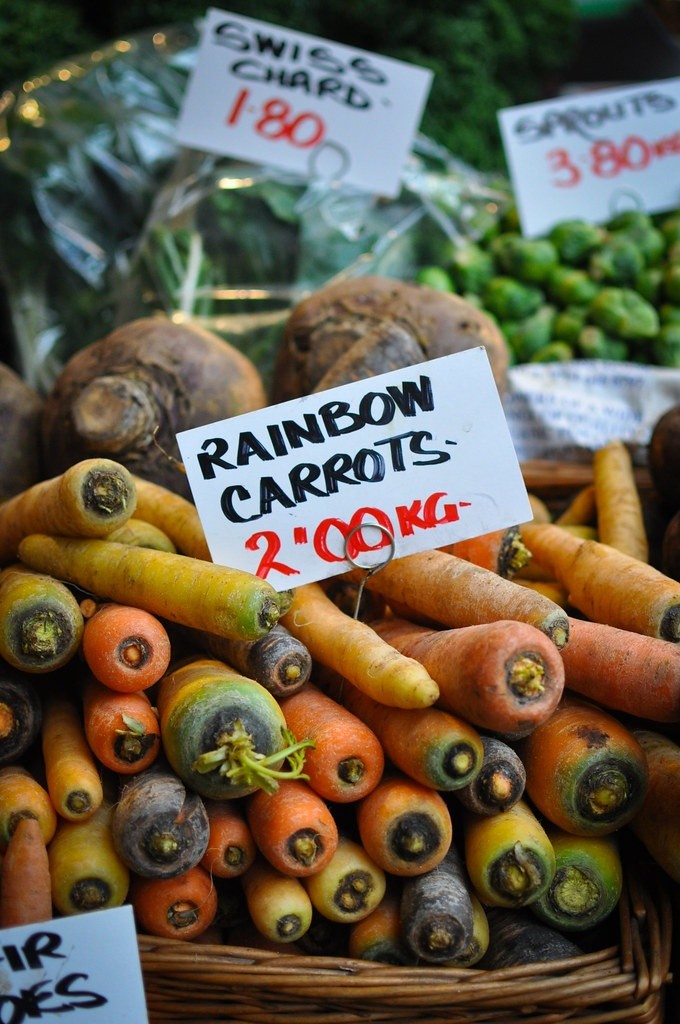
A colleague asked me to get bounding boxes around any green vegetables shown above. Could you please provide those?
[1,0,623,388]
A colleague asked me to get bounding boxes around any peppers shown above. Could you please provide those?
[411,198,680,379]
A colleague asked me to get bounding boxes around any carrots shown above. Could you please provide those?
[0,441,680,967]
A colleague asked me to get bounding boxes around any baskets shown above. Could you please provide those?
[137,878,672,1024]
[519,459,656,524]
